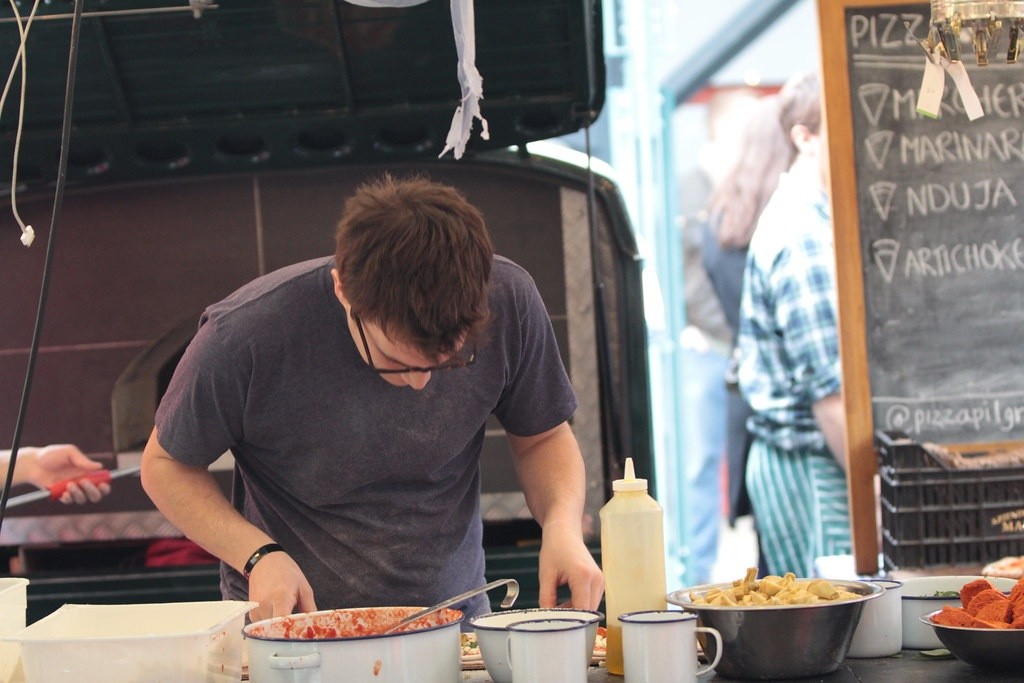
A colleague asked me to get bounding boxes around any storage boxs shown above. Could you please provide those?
[874,428,1024,572]
[0,599,259,683]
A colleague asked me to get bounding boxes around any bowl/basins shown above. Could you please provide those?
[1,601,262,683]
[240,606,465,683]
[0,576,30,683]
[468,607,605,683]
[918,609,1024,676]
[664,578,886,680]
[899,575,1018,648]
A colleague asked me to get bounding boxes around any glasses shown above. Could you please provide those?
[354,312,477,374]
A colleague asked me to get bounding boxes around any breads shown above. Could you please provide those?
[930,576,1024,632]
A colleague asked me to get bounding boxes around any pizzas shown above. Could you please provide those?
[593,627,607,657]
[460,634,483,660]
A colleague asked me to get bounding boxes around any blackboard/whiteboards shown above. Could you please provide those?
[819,0,1023,444]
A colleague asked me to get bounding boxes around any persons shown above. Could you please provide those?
[1,443,110,504]
[141,179,605,633]
[697,76,853,583]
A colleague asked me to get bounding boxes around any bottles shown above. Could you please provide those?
[599,457,667,675]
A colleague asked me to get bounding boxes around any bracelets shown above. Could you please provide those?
[243,544,286,580]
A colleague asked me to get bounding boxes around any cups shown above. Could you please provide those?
[505,618,589,683]
[617,610,723,683]
[846,579,904,657]
